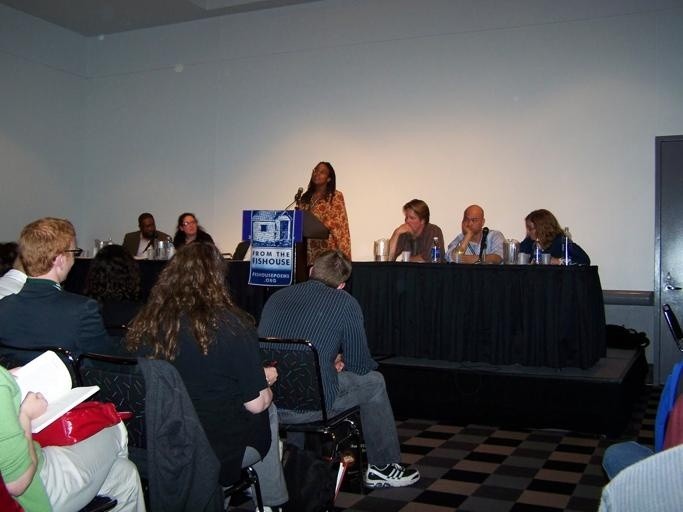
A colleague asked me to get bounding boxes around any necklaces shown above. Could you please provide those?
[311,198,319,208]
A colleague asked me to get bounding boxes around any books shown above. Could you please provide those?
[11,349,100,435]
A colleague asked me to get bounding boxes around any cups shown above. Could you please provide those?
[402,251,411,262]
[518,252,531,265]
[541,254,551,264]
[147,249,156,260]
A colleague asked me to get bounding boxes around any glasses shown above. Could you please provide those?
[63,247,83,257]
[180,220,196,226]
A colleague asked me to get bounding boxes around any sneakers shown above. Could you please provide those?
[362,463,420,489]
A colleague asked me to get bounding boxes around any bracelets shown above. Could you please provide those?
[266,377,271,384]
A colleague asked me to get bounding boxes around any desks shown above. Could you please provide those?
[0,259,607,368]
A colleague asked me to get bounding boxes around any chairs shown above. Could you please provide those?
[257,336,365,491]
[1,342,83,391]
[663,304,683,352]
[220,240,250,261]
[72,354,152,511]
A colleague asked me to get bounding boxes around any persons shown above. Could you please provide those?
[448,206,507,265]
[520,209,590,267]
[123,213,172,259]
[388,199,445,263]
[601,364,681,512]
[294,161,351,265]
[0,242,26,299]
[256,250,421,488]
[0,218,112,363]
[84,244,145,328]
[125,244,290,512]
[0,366,146,512]
[173,213,215,250]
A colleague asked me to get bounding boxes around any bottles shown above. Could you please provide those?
[533,238,542,264]
[455,243,465,264]
[166,237,173,260]
[157,240,165,261]
[430,237,441,263]
[562,227,572,265]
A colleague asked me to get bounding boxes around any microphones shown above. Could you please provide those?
[143,233,158,253]
[479,227,489,258]
[296,187,303,202]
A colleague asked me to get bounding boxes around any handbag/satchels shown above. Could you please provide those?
[30,401,132,448]
